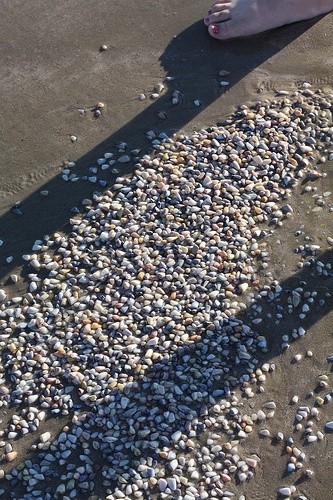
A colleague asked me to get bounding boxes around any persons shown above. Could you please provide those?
[203,0,333,40]
